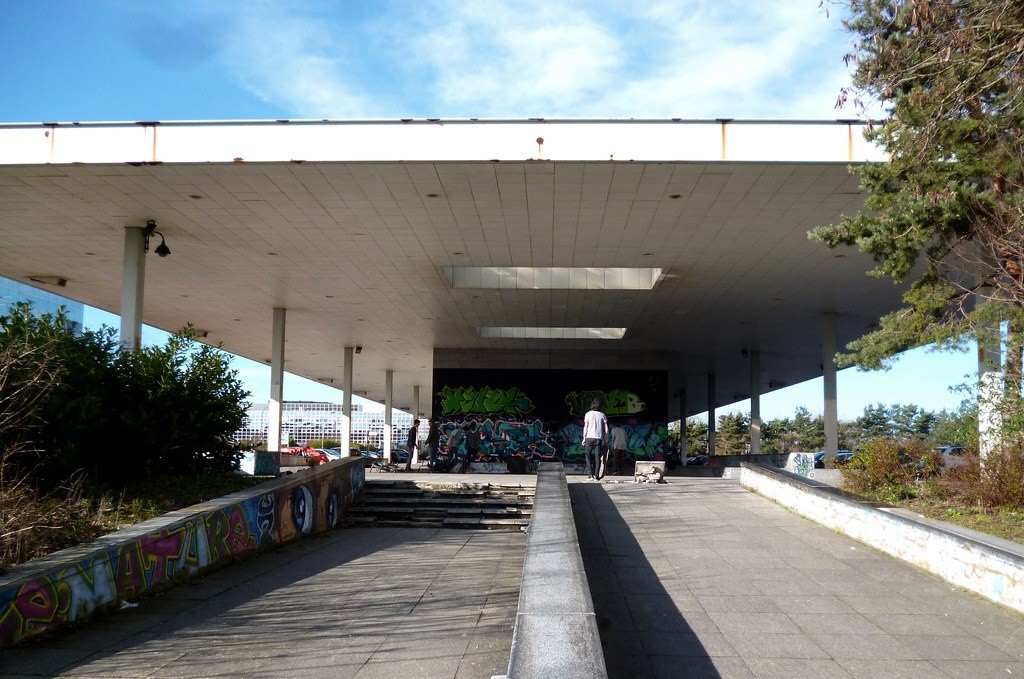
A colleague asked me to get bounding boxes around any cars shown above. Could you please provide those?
[813,450,855,468]
[685,454,710,466]
[280,447,409,465]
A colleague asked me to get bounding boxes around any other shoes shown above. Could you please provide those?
[595,479,599,482]
[588,476,594,480]
[612,472,617,475]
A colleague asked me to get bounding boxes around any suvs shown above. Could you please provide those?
[914,443,972,477]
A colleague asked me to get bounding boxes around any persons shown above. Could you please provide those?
[404,419,420,471]
[611,423,628,476]
[424,418,439,472]
[446,421,479,473]
[582,398,609,480]
